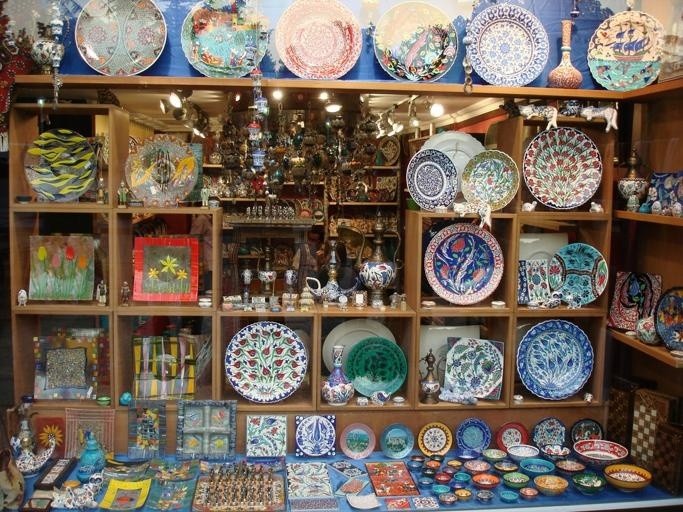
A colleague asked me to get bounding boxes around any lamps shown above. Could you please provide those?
[320,88,343,116]
[373,92,448,138]
[157,88,215,141]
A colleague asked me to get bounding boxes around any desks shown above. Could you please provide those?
[9,445,682,511]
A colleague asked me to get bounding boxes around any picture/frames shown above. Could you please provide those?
[171,397,238,462]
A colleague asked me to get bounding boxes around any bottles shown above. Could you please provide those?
[32,24,66,74]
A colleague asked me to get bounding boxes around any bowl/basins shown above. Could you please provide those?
[242,412,654,511]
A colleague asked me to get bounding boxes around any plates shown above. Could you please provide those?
[179,3,268,78]
[518,242,609,307]
[133,334,197,401]
[585,10,665,92]
[23,127,100,204]
[443,336,506,402]
[371,0,460,83]
[274,1,365,82]
[24,231,99,308]
[31,333,102,401]
[425,222,505,307]
[465,3,550,86]
[220,317,407,407]
[125,134,201,209]
[523,125,606,214]
[611,250,683,352]
[517,320,593,400]
[401,125,521,214]
[73,1,171,76]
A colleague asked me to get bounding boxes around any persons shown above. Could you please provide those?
[117,182,129,205]
[96,280,107,304]
[201,185,209,207]
[190,196,220,290]
[121,282,130,304]
[292,231,356,333]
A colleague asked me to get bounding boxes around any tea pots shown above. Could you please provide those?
[224,219,408,310]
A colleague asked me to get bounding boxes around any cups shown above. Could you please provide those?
[96,396,112,407]
[21,396,35,404]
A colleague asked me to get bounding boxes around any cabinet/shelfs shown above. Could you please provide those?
[607,76,683,490]
[2,70,616,451]
